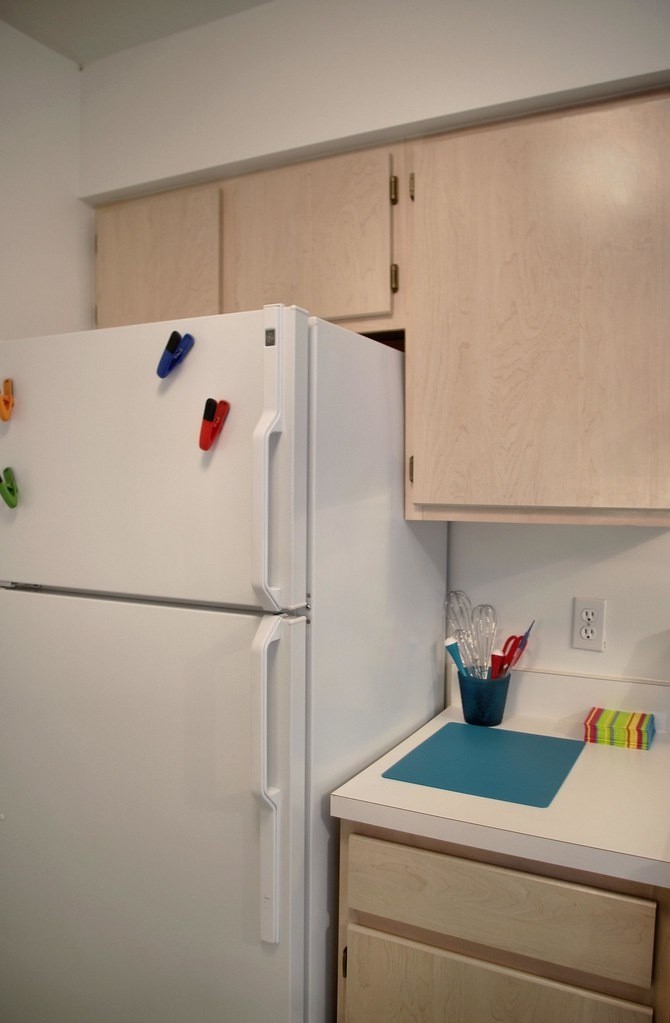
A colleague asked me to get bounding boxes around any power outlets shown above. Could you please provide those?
[571,598,607,652]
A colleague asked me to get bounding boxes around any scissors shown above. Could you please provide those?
[497,634,528,679]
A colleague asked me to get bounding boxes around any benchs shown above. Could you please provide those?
[342,832,658,1023]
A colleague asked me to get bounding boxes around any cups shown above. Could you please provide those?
[458,667,511,726]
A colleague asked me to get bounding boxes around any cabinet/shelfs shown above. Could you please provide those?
[93,149,397,331]
[410,100,670,510]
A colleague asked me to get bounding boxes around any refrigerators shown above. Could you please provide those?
[0,305,446,1022]
